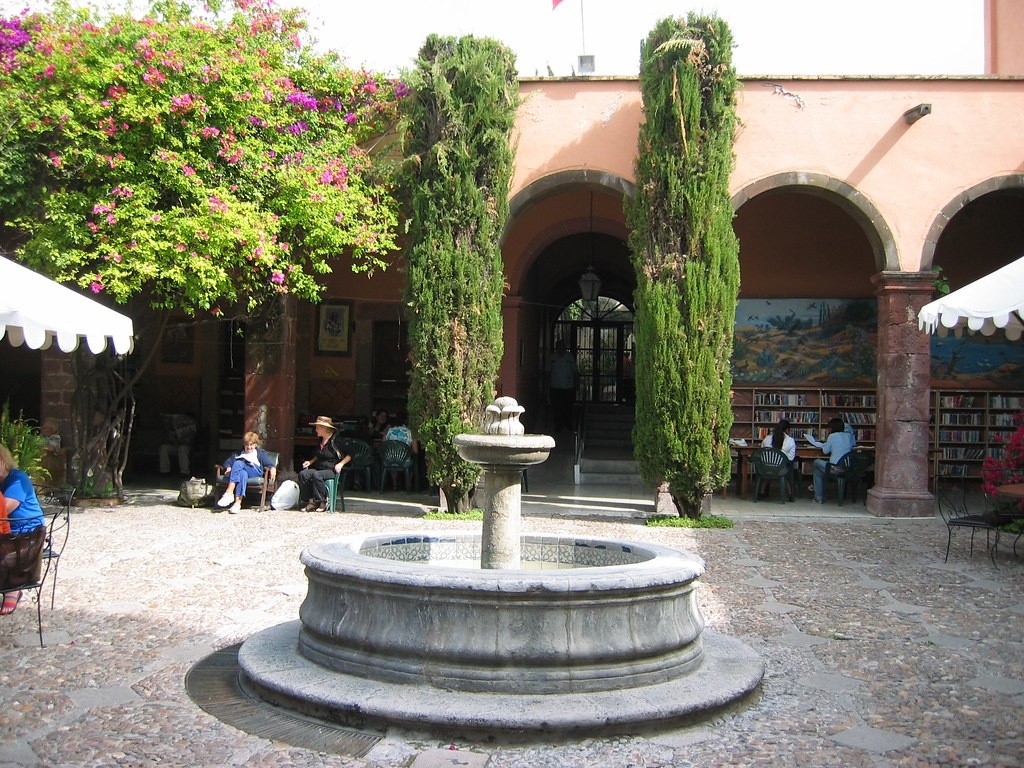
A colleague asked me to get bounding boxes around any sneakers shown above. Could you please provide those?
[808,485,815,492]
[229,503,242,514]
[812,498,822,505]
[217,492,235,507]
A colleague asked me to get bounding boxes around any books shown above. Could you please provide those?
[988,395,1024,461]
[754,391,877,441]
[938,395,986,476]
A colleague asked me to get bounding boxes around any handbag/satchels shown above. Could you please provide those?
[177,477,216,508]
[270,480,299,510]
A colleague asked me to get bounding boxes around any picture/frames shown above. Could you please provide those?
[160,315,194,365]
[313,297,355,358]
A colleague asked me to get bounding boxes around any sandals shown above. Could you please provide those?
[301,502,316,512]
[316,499,329,512]
[353,483,361,491]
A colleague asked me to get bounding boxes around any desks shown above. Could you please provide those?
[295,433,425,493]
[731,443,877,499]
[995,483,1024,498]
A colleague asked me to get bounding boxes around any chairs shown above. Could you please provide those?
[0,511,58,649]
[749,448,797,505]
[822,448,875,507]
[170,421,211,476]
[339,436,375,493]
[32,483,77,610]
[297,459,354,513]
[213,450,281,511]
[375,440,421,495]
[938,472,1022,571]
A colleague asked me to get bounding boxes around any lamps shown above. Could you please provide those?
[577,189,604,302]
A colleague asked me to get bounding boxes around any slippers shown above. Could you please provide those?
[0,590,22,616]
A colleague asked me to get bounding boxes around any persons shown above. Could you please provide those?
[217,431,276,514]
[40,416,61,455]
[615,351,636,406]
[298,416,355,512]
[547,340,580,432]
[758,420,796,502]
[0,443,46,615]
[158,398,200,478]
[803,417,857,504]
[0,490,11,534]
[89,409,117,467]
[353,409,418,491]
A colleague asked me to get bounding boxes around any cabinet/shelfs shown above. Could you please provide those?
[935,389,1024,495]
[730,386,938,496]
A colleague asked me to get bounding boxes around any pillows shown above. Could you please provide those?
[0,525,47,590]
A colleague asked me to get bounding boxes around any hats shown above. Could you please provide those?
[308,416,337,429]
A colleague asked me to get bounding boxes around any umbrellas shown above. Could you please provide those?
[0,256,134,355]
[916,255,1024,341]
